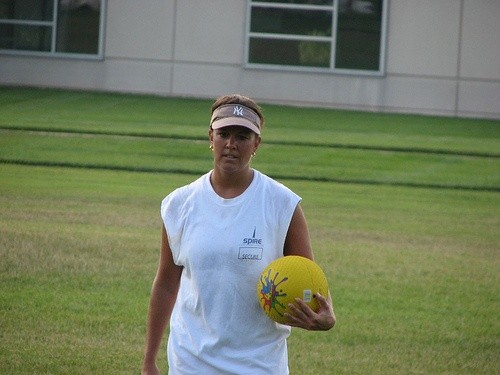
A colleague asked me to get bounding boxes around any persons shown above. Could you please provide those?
[142,95,336,375]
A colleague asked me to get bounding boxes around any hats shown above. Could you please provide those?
[210,104,261,135]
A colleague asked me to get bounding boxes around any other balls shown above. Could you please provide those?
[257,255,330,326]
